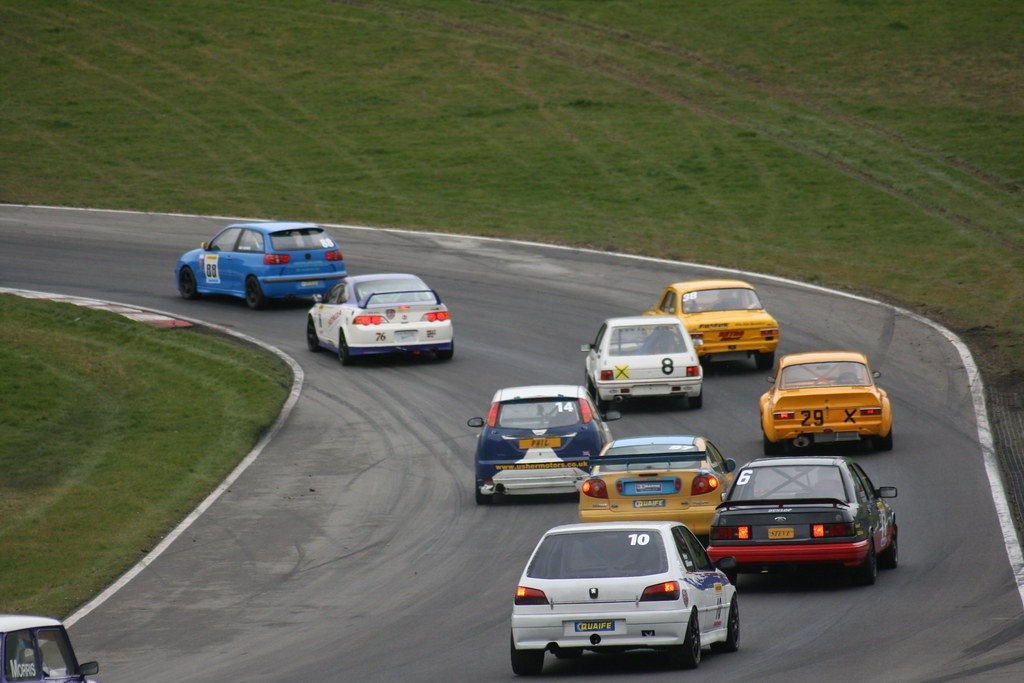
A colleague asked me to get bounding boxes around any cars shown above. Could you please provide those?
[509,520,740,674]
[576,435,734,556]
[580,315,706,411]
[641,278,779,368]
[702,456,898,585]
[305,270,455,367]
[175,221,347,309]
[466,385,624,504]
[758,350,895,454]
